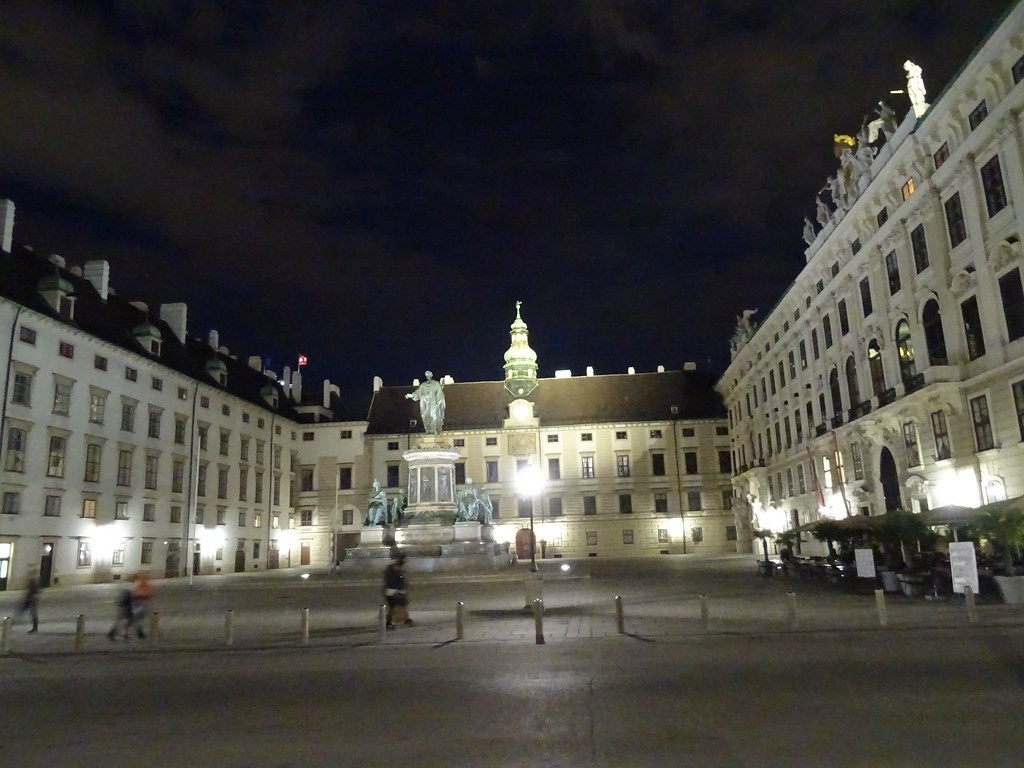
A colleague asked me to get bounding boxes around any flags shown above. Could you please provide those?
[299,355,307,365]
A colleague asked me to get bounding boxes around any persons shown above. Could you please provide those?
[11,569,40,634]
[458,475,494,527]
[404,370,446,435]
[803,59,930,246]
[390,489,405,523]
[729,309,758,361]
[385,553,414,629]
[109,579,154,640]
[367,479,388,525]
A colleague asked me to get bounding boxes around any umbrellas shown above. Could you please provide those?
[783,495,1024,563]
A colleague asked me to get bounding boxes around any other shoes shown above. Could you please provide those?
[386,622,396,629]
[108,628,117,641]
[137,630,146,639]
[404,619,413,627]
[28,624,38,633]
